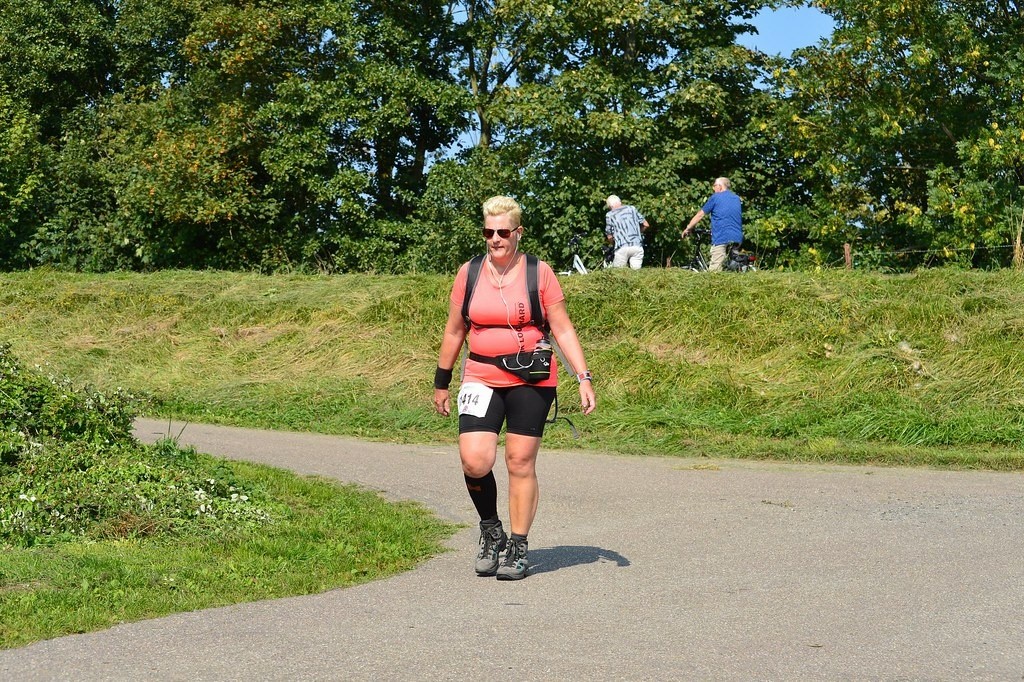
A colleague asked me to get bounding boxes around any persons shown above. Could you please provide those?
[605,194,649,270]
[434,196,596,580]
[682,177,743,271]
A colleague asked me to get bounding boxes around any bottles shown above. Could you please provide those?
[533,336,552,350]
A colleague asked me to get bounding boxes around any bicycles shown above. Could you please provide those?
[682,228,759,274]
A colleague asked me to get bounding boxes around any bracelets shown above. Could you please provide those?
[576,370,592,385]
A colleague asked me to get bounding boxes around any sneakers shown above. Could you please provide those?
[475,521,508,574]
[496,538,527,580]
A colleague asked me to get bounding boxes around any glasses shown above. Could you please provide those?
[482,226,519,238]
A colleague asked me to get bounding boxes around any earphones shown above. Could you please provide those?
[516,232,520,241]
